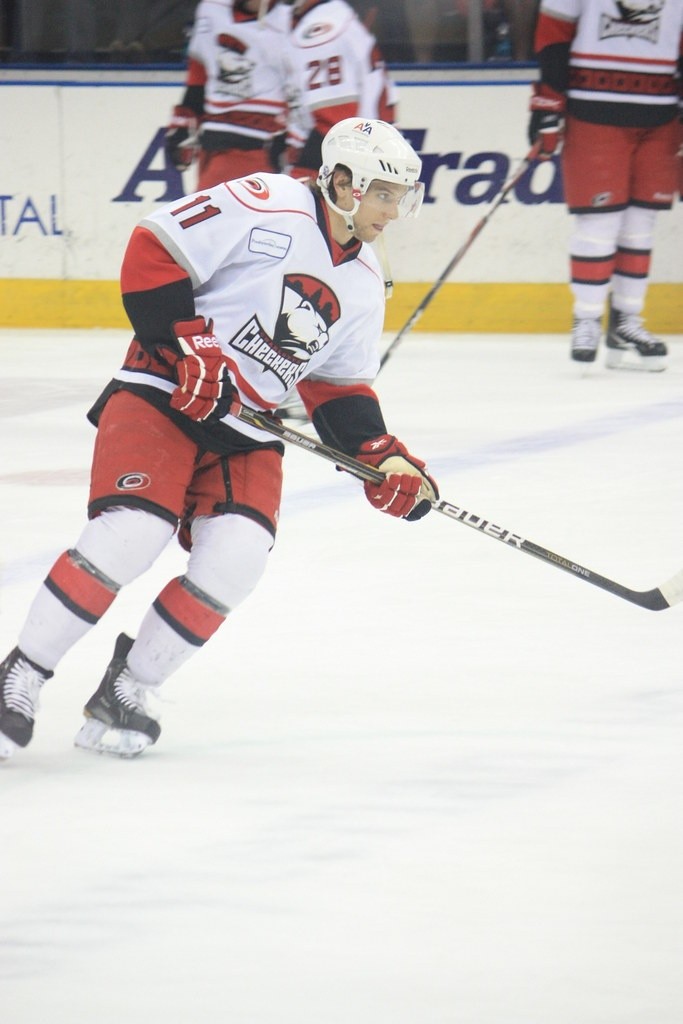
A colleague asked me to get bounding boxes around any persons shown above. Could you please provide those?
[522,0,683,372]
[164,0,397,420]
[0,118,439,764]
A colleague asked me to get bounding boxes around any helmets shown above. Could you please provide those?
[315,117,422,198]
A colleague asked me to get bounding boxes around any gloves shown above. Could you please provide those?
[155,315,233,430]
[163,105,199,173]
[526,78,567,156]
[336,434,440,522]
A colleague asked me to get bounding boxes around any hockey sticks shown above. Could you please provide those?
[376,138,544,378]
[229,399,683,612]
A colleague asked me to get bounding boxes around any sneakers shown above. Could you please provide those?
[74,632,160,758]
[0,646,54,756]
[571,315,604,381]
[603,291,667,372]
